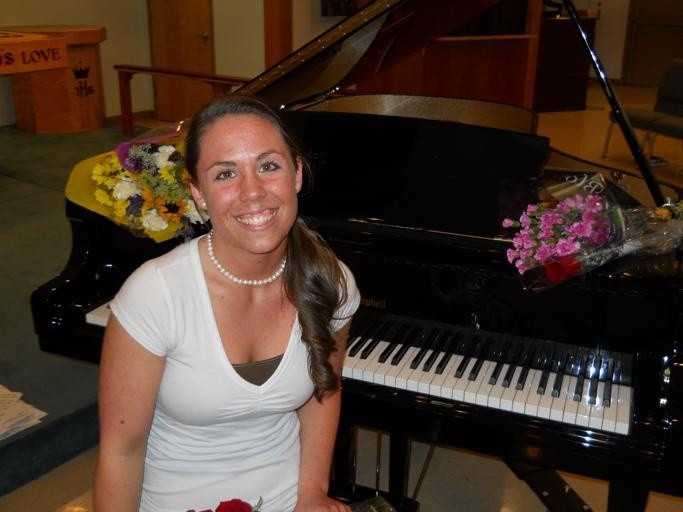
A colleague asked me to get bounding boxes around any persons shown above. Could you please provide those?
[94,94,351,512]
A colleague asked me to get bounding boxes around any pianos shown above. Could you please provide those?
[29,2,682,512]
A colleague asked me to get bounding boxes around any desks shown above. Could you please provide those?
[0,31,68,76]
[3,26,108,132]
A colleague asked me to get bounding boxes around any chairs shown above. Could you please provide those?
[603,60,683,163]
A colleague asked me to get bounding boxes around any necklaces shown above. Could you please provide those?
[207,229,288,285]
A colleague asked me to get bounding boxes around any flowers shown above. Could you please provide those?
[500,175,683,291]
[92,112,211,233]
